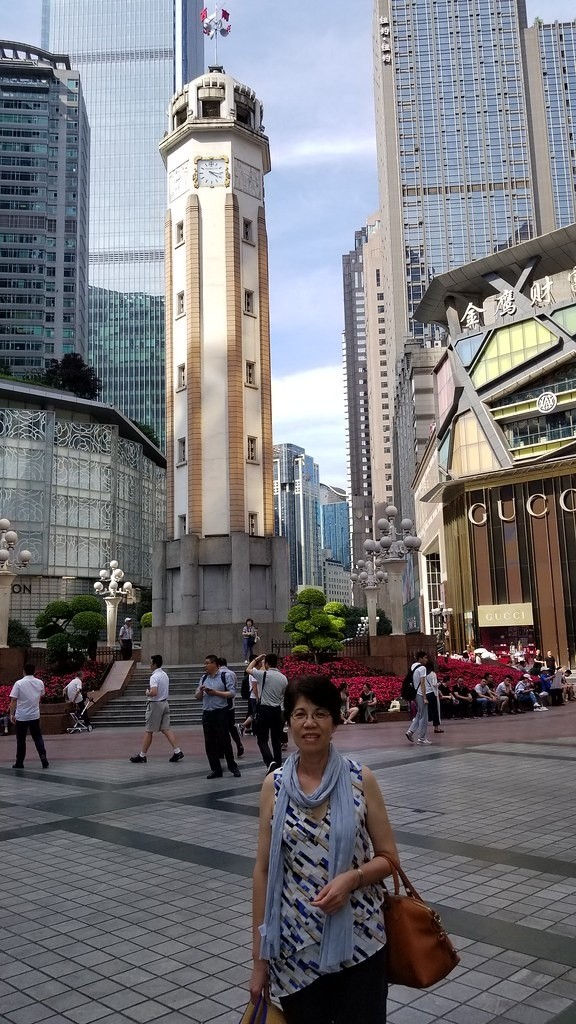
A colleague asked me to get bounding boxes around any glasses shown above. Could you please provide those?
[290,708,330,721]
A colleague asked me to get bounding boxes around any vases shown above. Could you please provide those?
[0,718,7,736]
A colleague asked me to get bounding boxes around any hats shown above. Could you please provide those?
[125,618,132,622]
[240,996,286,1024]
[540,667,550,671]
[523,673,533,681]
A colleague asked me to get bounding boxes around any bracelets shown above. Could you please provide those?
[357,869,364,887]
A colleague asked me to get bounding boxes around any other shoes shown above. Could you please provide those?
[344,720,356,725]
[234,770,241,777]
[265,761,277,776]
[245,727,253,735]
[434,730,444,733]
[540,706,548,711]
[237,746,245,758]
[244,660,248,663]
[207,771,224,779]
[238,724,245,738]
[13,764,24,768]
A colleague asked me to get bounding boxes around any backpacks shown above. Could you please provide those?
[241,674,253,700]
[400,664,421,701]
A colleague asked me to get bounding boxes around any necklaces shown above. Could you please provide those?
[297,804,328,844]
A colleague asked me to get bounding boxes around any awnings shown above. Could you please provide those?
[478,602,533,627]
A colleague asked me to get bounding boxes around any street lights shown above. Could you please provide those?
[350,553,389,636]
[93,559,132,646]
[377,505,422,635]
[431,601,453,643]
[0,519,32,650]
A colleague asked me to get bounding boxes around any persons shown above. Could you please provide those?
[474,678,496,716]
[195,655,244,778]
[461,653,471,662]
[439,676,460,719]
[475,654,482,664]
[496,666,576,713]
[242,619,256,663]
[119,617,133,660]
[453,676,475,719]
[236,654,288,777]
[524,649,530,664]
[129,655,184,762]
[9,664,49,769]
[510,642,515,654]
[359,683,377,723]
[490,650,497,660]
[543,650,555,675]
[63,671,96,730]
[248,677,401,1024]
[485,673,508,715]
[533,649,543,668]
[425,661,445,732]
[338,683,359,724]
[405,651,432,746]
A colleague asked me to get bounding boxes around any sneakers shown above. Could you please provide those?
[405,730,414,742]
[533,703,541,707]
[129,754,147,763]
[417,739,432,745]
[534,708,542,712]
[169,751,184,762]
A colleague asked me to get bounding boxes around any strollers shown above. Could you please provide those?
[64,696,96,734]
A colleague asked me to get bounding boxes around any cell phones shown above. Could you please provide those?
[202,686,211,692]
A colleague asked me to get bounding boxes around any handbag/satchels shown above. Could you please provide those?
[371,853,461,988]
